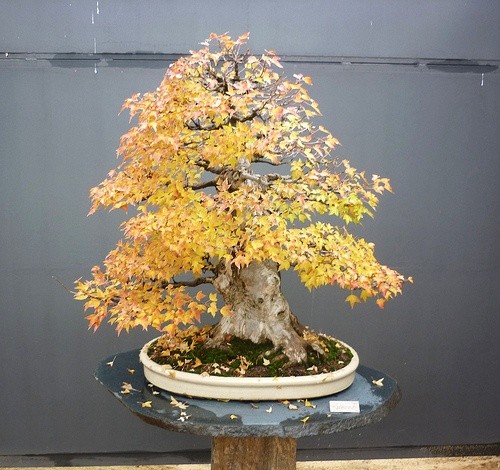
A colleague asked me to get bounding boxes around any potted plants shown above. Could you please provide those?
[70,26,415,401]
